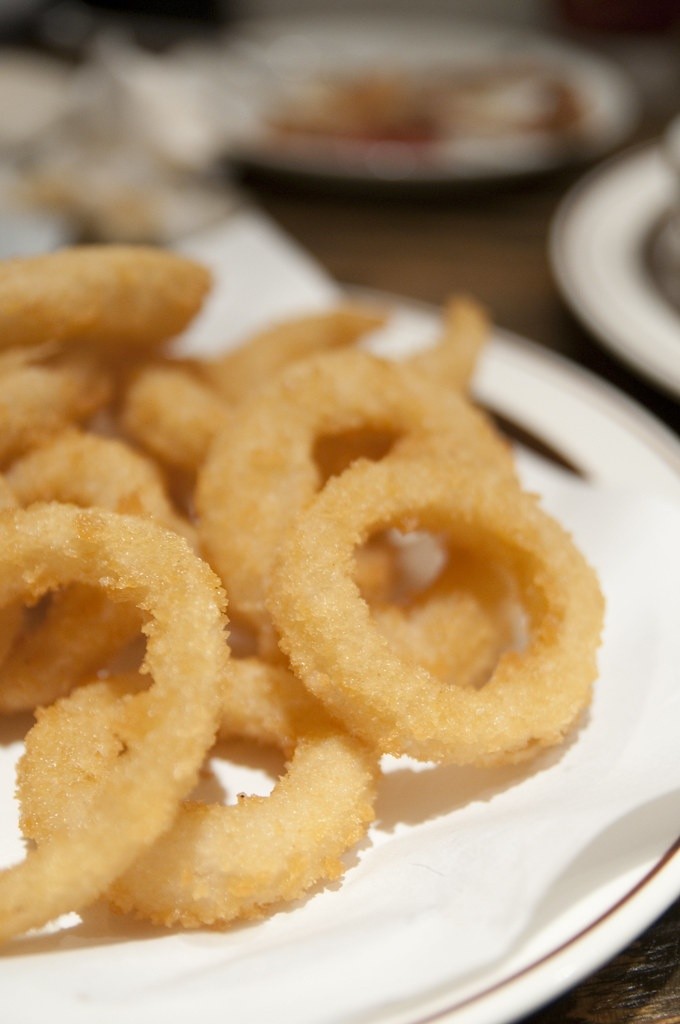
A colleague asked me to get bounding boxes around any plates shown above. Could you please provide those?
[0,294,680,1024]
[547,143,680,392]
[176,28,632,182]
